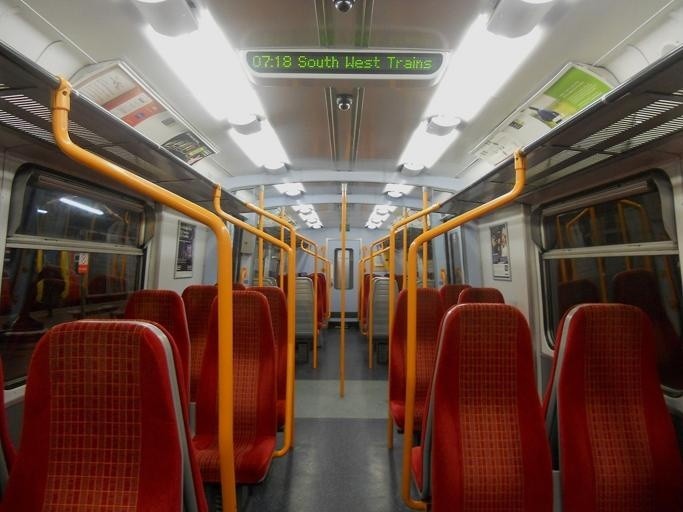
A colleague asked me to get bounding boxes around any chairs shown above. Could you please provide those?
[0,284,288,511]
[391,285,683,512]
[358,274,434,365]
[253,273,326,366]
